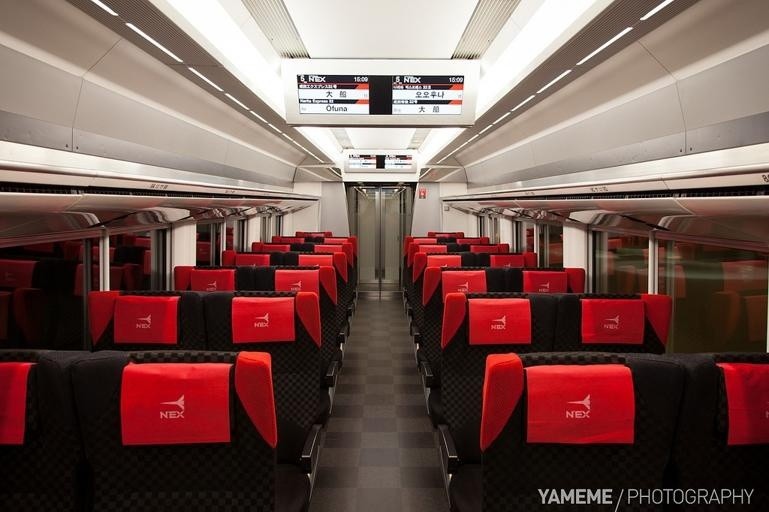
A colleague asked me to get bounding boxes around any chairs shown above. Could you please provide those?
[407,243,459,336]
[203,292,338,433]
[284,251,354,343]
[447,237,489,243]
[313,235,357,244]
[272,237,312,244]
[70,352,323,511]
[476,252,537,267]
[662,352,769,512]
[174,266,255,290]
[88,291,207,352]
[421,267,505,430]
[553,293,672,351]
[297,232,333,236]
[252,243,300,252]
[460,243,509,253]
[428,232,464,237]
[300,244,356,317]
[0,349,91,512]
[403,237,446,316]
[506,267,585,293]
[254,267,348,371]
[438,293,556,511]
[222,251,284,267]
[412,252,475,373]
[480,353,685,511]
